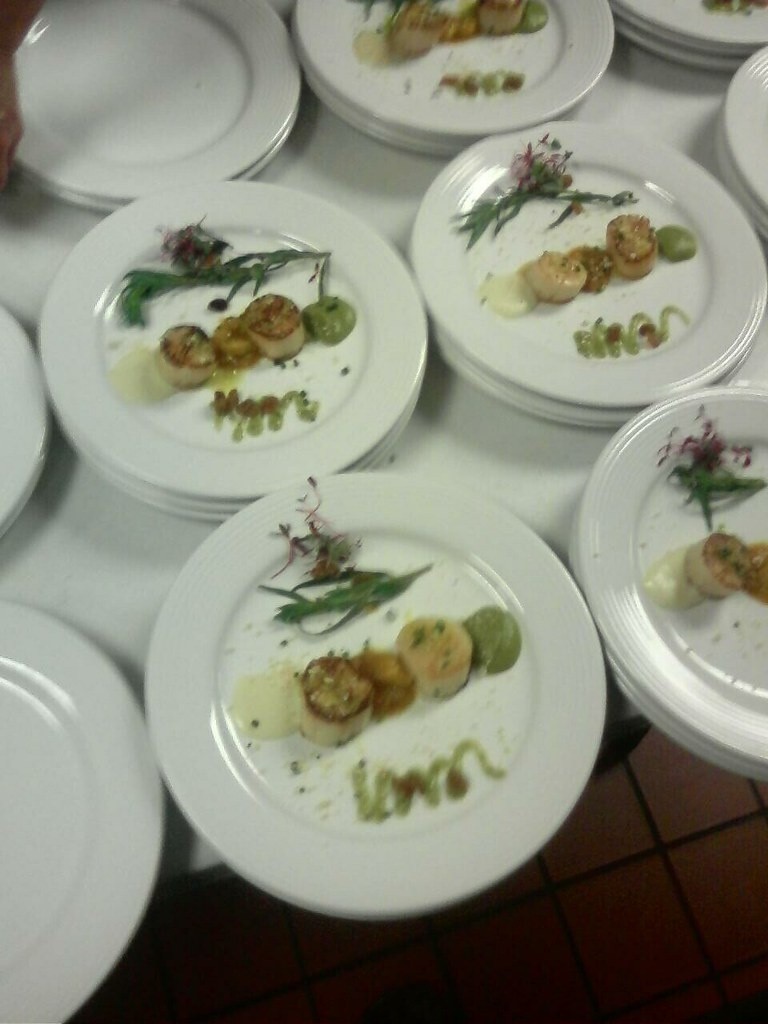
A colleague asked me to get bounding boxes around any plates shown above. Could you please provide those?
[406,120,768,428]
[0,300,50,540]
[144,472,607,920]
[713,44,768,240]
[289,0,616,157]
[570,387,768,783]
[608,0,768,73]
[10,0,301,211]
[0,600,165,1024]
[36,181,429,523]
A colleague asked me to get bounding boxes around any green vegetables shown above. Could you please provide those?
[117,144,767,636]
